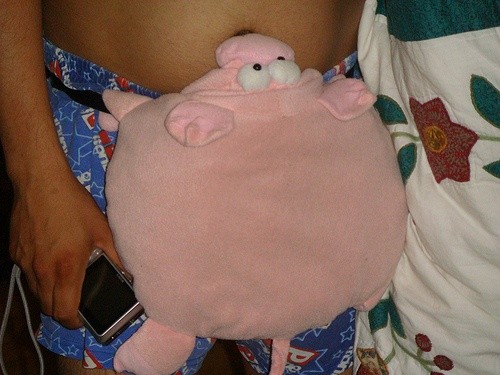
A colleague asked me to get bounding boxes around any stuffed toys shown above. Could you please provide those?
[97,30,410,375]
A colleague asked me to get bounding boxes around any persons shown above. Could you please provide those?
[1,0,367,375]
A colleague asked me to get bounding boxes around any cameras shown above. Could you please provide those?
[76,248,144,345]
